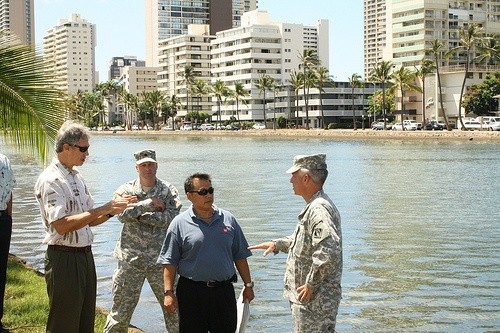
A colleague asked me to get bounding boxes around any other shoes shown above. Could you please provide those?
[0,324,9,333]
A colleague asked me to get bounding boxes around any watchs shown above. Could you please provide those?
[163,290,174,295]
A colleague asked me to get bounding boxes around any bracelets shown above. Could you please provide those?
[244,281,254,289]
[106,213,114,218]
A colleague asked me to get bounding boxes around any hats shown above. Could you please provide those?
[284,153,327,174]
[134,149,157,165]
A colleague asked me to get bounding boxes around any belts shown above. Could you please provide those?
[180,274,233,288]
[55,246,91,253]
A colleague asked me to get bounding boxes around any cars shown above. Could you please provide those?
[180,123,266,131]
[131,125,154,131]
[461,116,500,131]
[110,125,125,131]
[371,119,423,131]
[164,125,174,131]
[424,120,456,131]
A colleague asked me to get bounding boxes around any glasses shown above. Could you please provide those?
[70,144,88,152]
[191,186,215,196]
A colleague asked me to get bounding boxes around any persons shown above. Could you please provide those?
[0,153,17,333]
[247,154,343,333]
[156,173,255,333]
[34,119,137,333]
[103,149,181,333]
